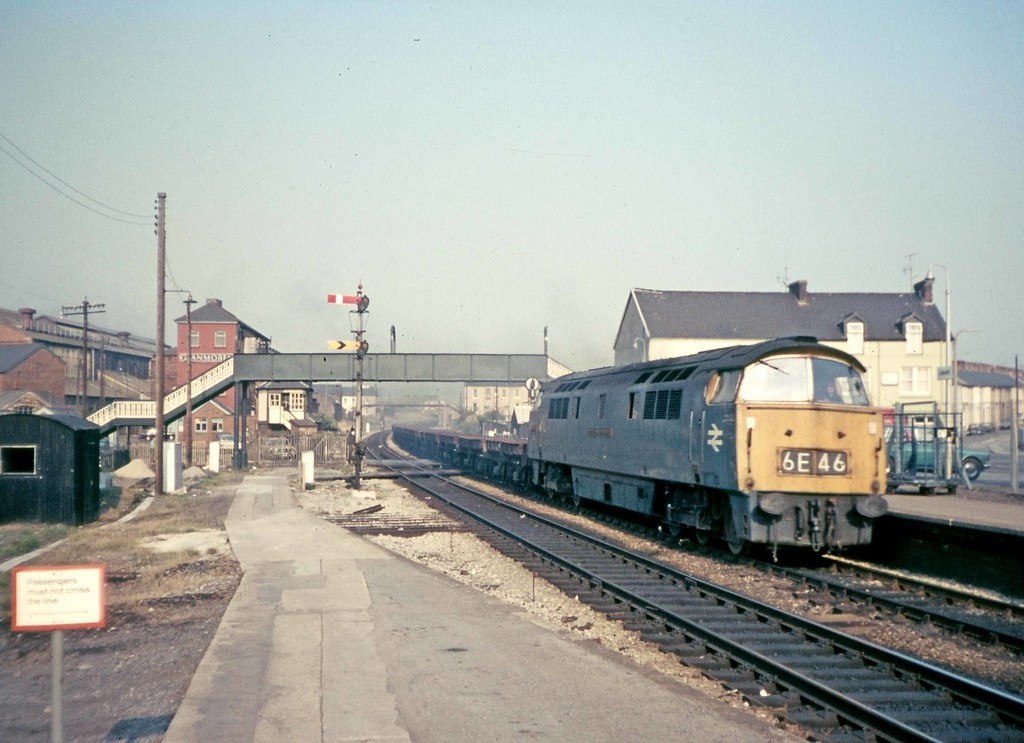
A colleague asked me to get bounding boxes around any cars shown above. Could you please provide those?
[966,421,1011,438]
[886,425,989,482]
[260,436,298,460]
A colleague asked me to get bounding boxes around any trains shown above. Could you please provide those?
[390,336,890,568]
